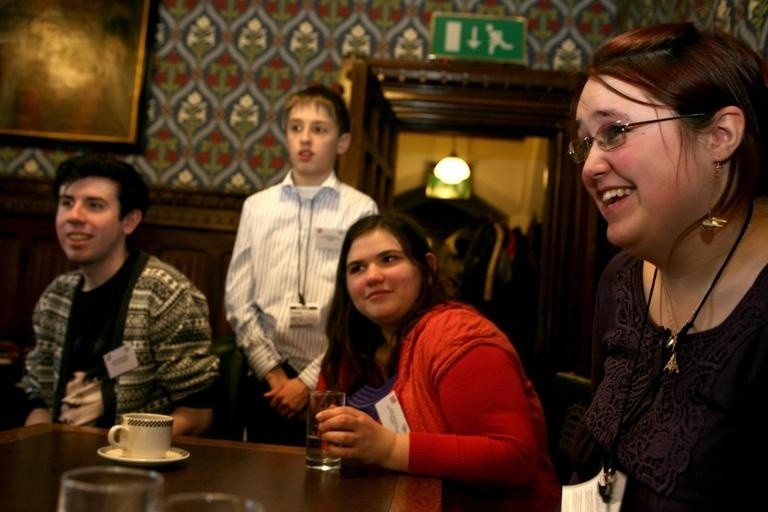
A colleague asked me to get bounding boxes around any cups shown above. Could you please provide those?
[107,412,174,460]
[305,390,348,473]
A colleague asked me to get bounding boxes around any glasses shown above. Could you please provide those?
[566,112,703,167]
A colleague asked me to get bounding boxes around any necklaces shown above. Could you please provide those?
[647,197,756,376]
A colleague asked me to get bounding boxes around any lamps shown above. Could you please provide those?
[434,117,472,186]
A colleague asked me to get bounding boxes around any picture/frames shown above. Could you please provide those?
[0,0,160,156]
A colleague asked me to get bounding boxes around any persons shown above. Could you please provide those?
[222,79,380,445]
[314,211,561,510]
[15,152,218,437]
[558,23,766,510]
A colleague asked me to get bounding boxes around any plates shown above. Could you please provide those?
[97,444,192,467]
